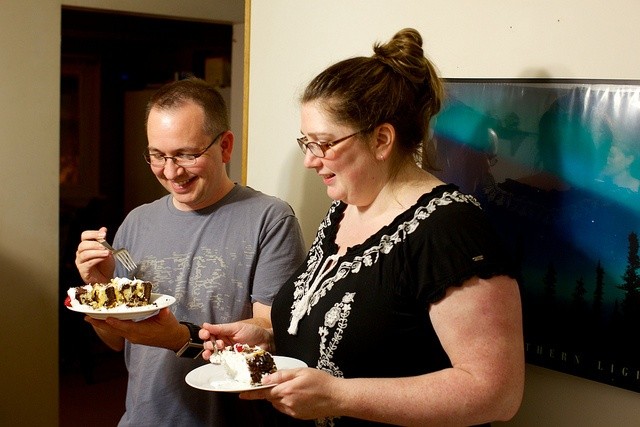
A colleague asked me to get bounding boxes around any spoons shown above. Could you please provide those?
[199,323,222,364]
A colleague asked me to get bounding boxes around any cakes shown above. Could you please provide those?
[217,341,276,384]
[64,276,153,311]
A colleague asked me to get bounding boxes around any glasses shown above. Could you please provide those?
[297,128,375,157]
[143,132,223,166]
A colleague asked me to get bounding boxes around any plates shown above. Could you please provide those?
[66,292,176,319]
[184,355,309,392]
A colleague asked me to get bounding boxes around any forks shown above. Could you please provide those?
[95,239,138,271]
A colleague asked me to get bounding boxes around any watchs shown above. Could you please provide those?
[172,320,207,360]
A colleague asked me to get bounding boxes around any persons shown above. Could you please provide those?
[195,24,526,425]
[476,93,639,369]
[434,119,501,194]
[72,76,308,426]
